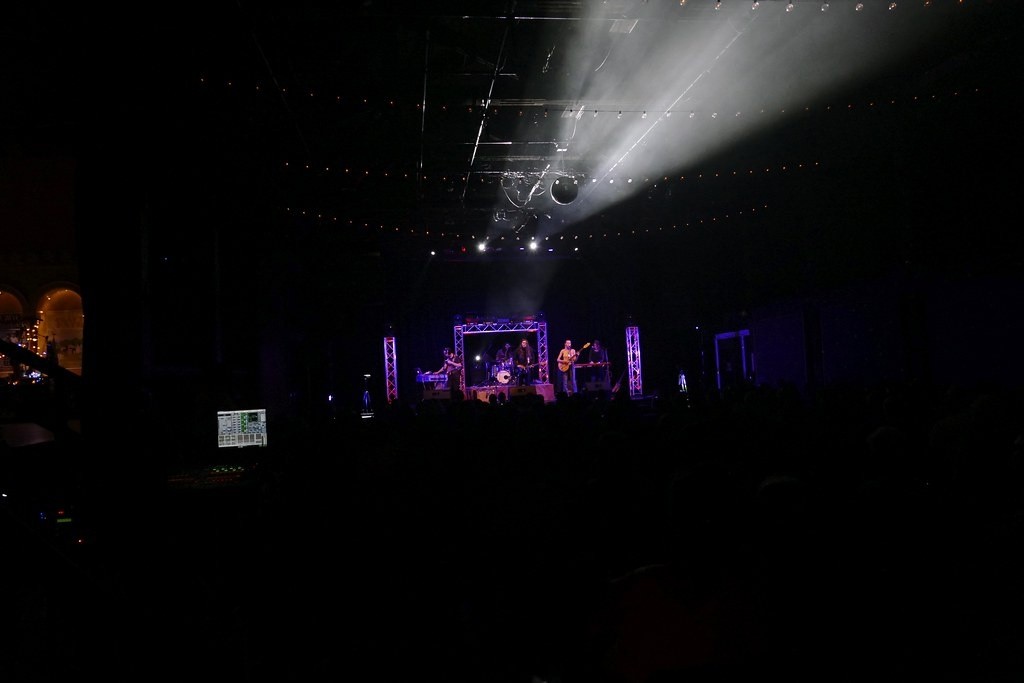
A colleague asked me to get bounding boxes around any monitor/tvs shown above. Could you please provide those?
[217,409,267,448]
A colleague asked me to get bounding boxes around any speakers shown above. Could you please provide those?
[424,390,450,400]
[581,382,612,400]
[508,386,537,401]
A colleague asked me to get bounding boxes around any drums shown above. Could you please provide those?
[492,364,500,377]
[496,370,512,384]
[501,358,514,370]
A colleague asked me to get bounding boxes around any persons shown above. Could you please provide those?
[512,338,534,392]
[433,348,462,375]
[557,340,580,395]
[495,343,513,362]
[589,340,604,386]
[9,334,20,374]
[0,391,1024,683]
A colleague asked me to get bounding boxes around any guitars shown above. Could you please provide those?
[558,343,590,371]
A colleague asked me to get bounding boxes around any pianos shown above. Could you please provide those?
[416,373,448,383]
[574,363,609,368]
